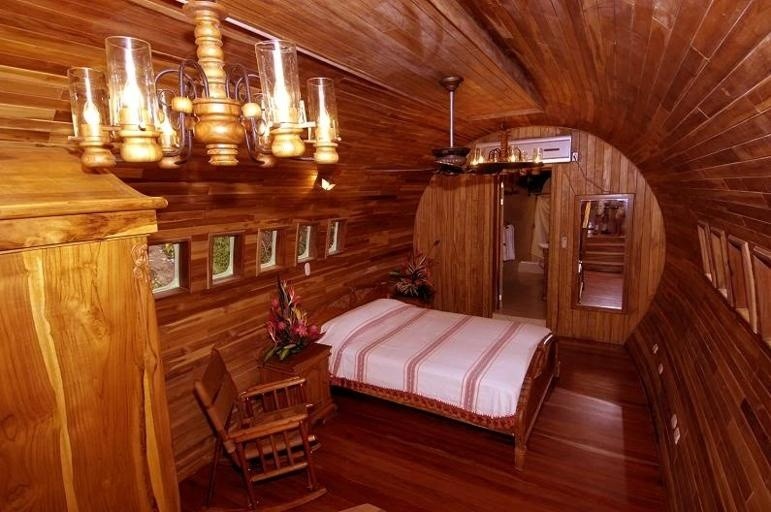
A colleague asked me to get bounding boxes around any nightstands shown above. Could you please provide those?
[256,343,337,426]
[388,294,438,308]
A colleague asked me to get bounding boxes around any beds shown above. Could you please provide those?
[311,298,564,471]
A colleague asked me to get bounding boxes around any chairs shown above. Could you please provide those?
[194,347,328,512]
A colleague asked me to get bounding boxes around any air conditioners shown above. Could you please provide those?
[475,134,572,165]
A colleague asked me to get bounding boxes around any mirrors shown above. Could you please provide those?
[570,192,635,316]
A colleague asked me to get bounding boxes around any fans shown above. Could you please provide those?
[364,73,545,177]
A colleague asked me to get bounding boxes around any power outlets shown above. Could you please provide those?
[571,152,578,162]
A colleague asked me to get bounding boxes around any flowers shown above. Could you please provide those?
[260,275,325,359]
[387,239,442,299]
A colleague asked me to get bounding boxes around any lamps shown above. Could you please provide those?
[321,178,336,191]
[68,1,340,168]
[467,125,545,177]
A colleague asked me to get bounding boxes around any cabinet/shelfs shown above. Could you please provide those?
[2,142,180,510]
